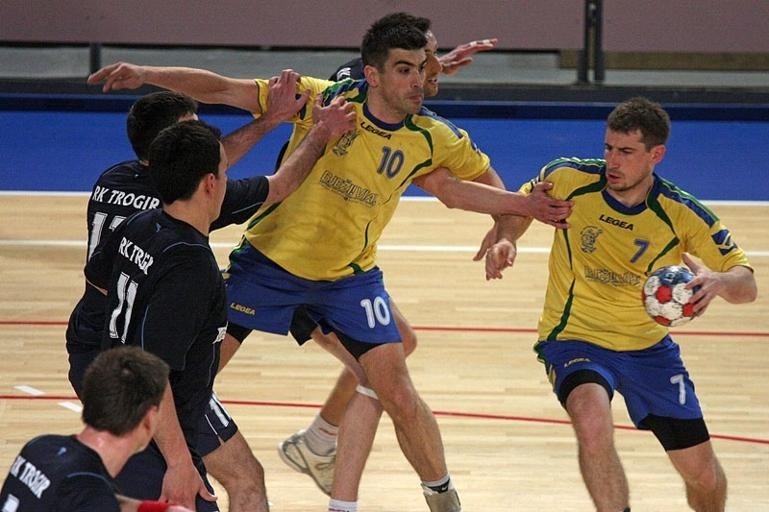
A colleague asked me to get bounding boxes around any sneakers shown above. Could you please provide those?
[278,426,345,499]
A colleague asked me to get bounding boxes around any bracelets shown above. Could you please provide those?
[137,500,170,512]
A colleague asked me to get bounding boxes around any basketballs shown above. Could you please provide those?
[641,265,704,327]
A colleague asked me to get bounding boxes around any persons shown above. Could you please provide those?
[1,341,172,511]
[482,95,760,512]
[81,18,506,511]
[262,11,578,500]
[81,122,272,512]
[63,64,359,400]
